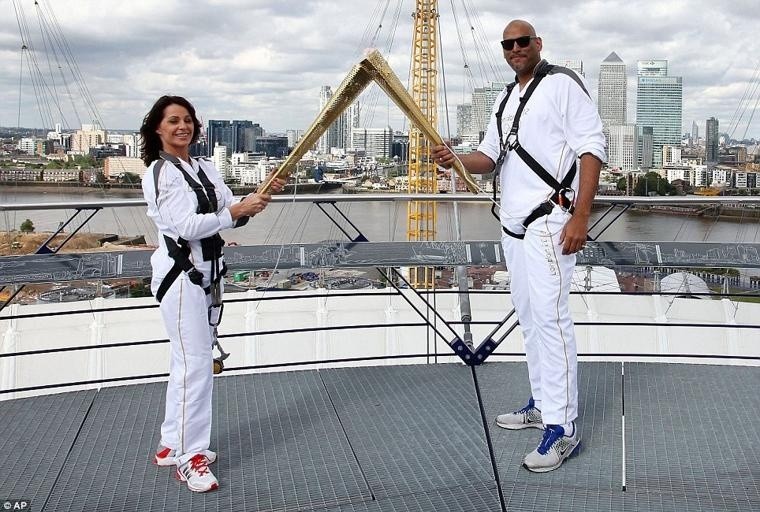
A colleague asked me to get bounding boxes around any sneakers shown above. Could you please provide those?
[495,397,581,474]
[155,445,220,493]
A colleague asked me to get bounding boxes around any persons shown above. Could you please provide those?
[430,18,609,475]
[137,95,293,493]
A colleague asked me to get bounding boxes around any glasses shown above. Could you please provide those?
[500,36,535,51]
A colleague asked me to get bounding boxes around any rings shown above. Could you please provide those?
[439,157,443,162]
[581,244,588,248]
[280,184,285,191]
